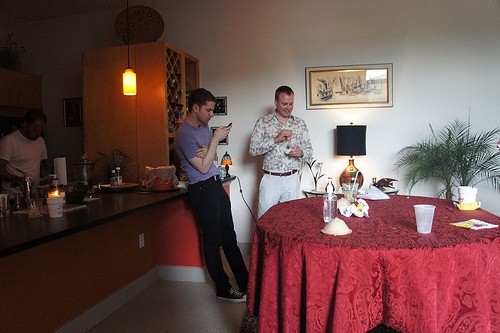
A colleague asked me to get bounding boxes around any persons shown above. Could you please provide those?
[173,87,249,302]
[0,108,54,198]
[247,86,313,222]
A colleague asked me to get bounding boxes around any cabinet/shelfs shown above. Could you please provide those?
[0,66,42,118]
[82,41,200,178]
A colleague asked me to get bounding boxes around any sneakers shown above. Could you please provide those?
[215,286,247,302]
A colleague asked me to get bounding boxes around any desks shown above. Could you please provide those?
[239,195,500,333]
[302,186,400,197]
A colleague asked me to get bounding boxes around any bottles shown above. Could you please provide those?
[116,167,122,185]
[110,170,117,185]
[322,177,338,223]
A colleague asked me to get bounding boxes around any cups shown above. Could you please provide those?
[0,194,8,211]
[413,204,436,234]
[30,188,43,218]
[342,183,359,206]
[47,198,63,218]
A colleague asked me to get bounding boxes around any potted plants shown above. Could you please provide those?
[0,31,28,73]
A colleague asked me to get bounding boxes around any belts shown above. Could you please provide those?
[188,174,219,189]
[264,169,297,176]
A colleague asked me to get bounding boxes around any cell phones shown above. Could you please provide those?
[226,123,232,127]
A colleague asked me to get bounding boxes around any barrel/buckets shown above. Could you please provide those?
[73,160,96,191]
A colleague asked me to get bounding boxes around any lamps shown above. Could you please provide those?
[122,0,136,95]
[336,123,367,188]
[220,151,232,178]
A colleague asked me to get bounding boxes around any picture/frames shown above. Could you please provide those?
[213,95,228,117]
[305,63,393,110]
[210,126,229,145]
[62,98,82,128]
